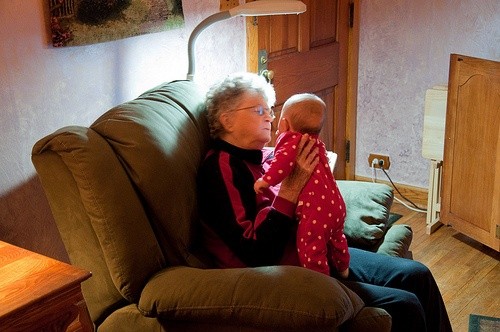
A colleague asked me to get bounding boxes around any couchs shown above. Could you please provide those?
[31,77,413,332]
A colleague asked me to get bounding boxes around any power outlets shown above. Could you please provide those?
[368,153,390,170]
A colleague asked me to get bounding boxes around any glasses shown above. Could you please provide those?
[227,103,276,120]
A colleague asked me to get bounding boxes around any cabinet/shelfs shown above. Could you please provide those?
[439,53,500,251]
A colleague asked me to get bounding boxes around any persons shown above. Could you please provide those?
[254,93,350,279]
[190,73,453,332]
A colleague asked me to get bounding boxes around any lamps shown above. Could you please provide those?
[186,0,307,83]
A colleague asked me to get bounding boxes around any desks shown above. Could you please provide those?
[0,241,94,332]
